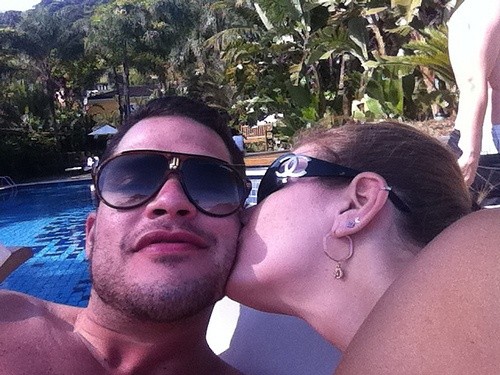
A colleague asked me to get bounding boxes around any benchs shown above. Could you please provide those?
[241,125,268,151]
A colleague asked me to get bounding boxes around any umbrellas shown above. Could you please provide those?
[88,125,117,136]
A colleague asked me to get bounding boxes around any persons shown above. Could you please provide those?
[446,0,500,208]
[230,128,247,156]
[226,123,500,375]
[0,96,252,375]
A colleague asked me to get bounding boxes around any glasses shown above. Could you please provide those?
[91,148,252,216]
[256,154,411,212]
